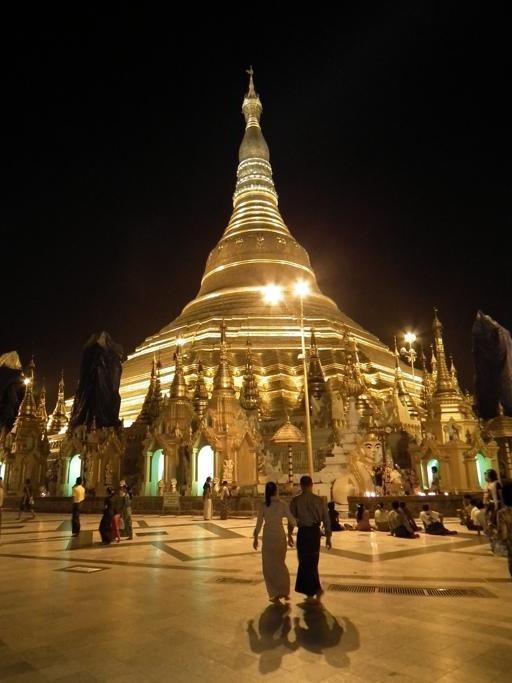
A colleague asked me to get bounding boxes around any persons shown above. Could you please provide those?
[1,477,5,526]
[321,501,345,531]
[202,483,216,520]
[70,476,86,537]
[349,430,387,497]
[221,451,234,479]
[15,478,38,520]
[346,463,511,575]
[109,485,124,545]
[97,487,115,546]
[218,480,232,520]
[287,474,334,603]
[118,481,134,540]
[252,482,299,603]
[202,476,214,499]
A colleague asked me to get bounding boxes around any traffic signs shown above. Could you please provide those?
[293,279,315,485]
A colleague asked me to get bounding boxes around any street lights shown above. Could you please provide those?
[405,330,417,394]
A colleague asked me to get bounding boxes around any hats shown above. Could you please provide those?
[107,487,114,492]
[120,480,126,486]
